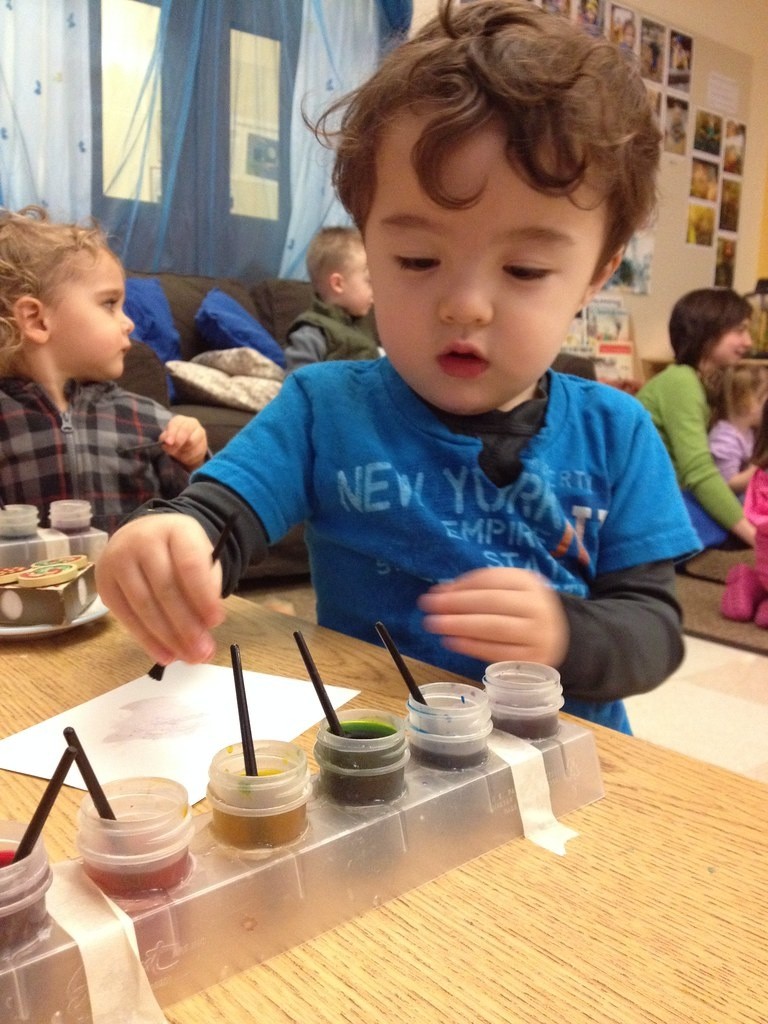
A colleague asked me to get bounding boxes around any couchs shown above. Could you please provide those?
[110,272,307,581]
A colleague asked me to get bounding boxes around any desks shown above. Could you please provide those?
[0,592,768,1024]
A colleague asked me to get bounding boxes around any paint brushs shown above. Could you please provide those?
[375,622,428,706]
[12,746,77,863]
[117,440,165,454]
[293,630,343,736]
[63,727,116,820]
[229,644,258,777]
[139,509,239,696]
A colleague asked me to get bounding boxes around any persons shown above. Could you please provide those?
[632,286,768,629]
[0,206,218,547]
[96,0,706,740]
[285,227,382,376]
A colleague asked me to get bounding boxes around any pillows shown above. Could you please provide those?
[123,278,289,414]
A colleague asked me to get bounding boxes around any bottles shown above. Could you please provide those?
[48,499,94,534]
[0,819,53,947]
[403,682,493,771]
[206,740,313,850]
[313,709,411,806]
[74,777,194,900]
[482,660,565,738]
[0,504,40,537]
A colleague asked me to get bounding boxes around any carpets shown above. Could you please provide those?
[672,548,768,655]
